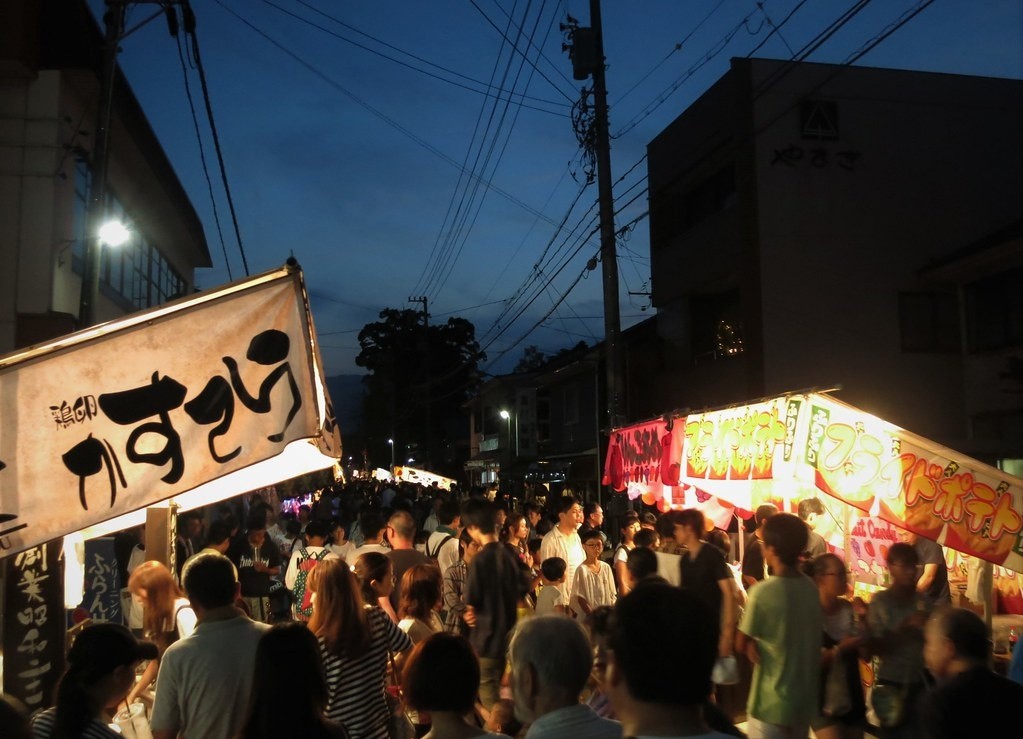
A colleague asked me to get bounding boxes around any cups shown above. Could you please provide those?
[112,703,154,739]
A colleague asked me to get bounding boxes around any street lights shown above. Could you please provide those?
[86,216,129,328]
[500,410,511,482]
[388,439,395,485]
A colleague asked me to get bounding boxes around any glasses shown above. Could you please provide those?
[823,572,851,579]
[891,563,920,571]
[584,543,604,549]
[384,523,394,534]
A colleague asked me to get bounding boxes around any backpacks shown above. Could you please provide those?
[292,547,331,617]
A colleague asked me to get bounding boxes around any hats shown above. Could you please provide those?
[68,623,158,678]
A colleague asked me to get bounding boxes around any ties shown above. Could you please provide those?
[185,539,192,557]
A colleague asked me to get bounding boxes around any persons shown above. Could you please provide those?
[26,476,1022,737]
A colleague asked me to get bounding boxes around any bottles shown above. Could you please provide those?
[1008,626,1018,652]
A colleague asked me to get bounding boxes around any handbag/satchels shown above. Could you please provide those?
[822,645,852,716]
[384,693,415,739]
[863,683,903,728]
[711,655,738,685]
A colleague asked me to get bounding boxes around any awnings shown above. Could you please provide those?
[601,389,1022,616]
[1,256,344,564]
[274,465,457,504]
